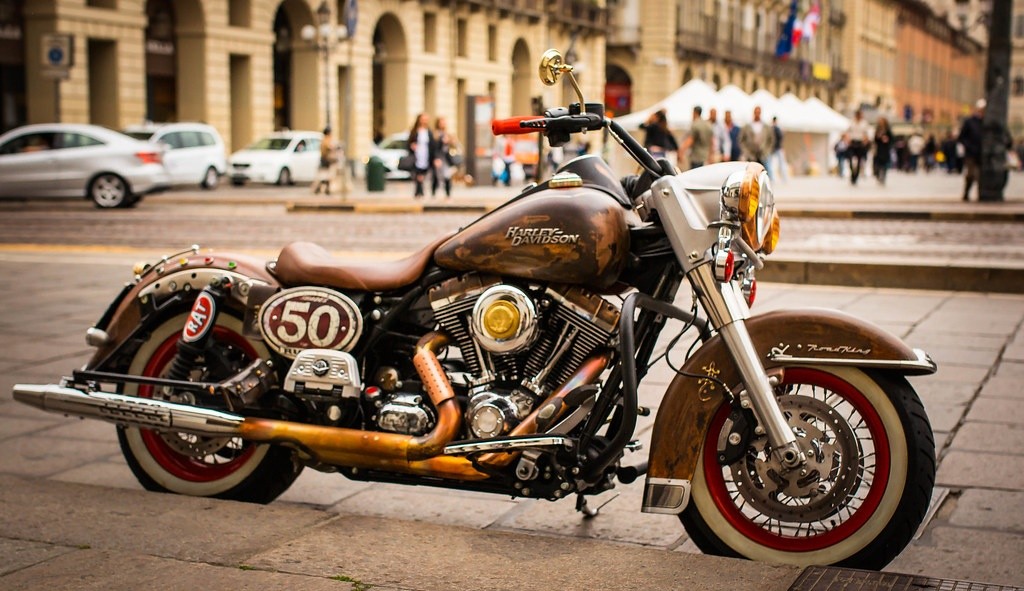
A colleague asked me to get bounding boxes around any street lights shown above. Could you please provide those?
[301,2,347,129]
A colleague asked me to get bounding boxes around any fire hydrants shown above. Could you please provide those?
[364,156,391,192]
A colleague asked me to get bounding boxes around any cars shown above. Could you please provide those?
[0,121,171,209]
[371,132,416,181]
[227,130,346,185]
[122,122,226,191]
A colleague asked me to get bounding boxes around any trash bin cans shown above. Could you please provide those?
[365,157,387,192]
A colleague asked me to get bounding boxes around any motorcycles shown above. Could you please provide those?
[13,49,939,570]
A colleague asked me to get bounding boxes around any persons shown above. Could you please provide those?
[873,116,895,184]
[406,113,459,197]
[895,131,965,174]
[834,134,848,178]
[844,111,870,184]
[313,127,343,194]
[679,106,786,180]
[959,98,1013,201]
[638,108,678,158]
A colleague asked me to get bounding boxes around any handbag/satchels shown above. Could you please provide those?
[397,156,417,171]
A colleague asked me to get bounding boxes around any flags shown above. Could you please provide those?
[776,0,821,60]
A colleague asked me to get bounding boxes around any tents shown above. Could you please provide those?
[607,75,874,182]
